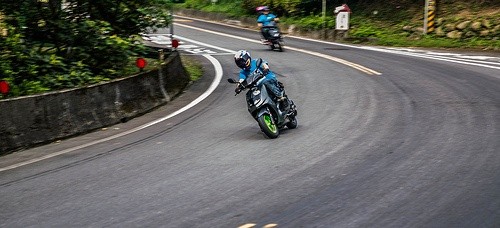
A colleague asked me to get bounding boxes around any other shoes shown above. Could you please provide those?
[279,98,289,112]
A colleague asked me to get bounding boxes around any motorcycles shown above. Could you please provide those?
[226,58,298,139]
[260,21,285,51]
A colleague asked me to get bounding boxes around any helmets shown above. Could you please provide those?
[234,49,252,70]
[256,6,270,11]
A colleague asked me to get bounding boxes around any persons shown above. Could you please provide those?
[234,49,290,111]
[256,7,285,46]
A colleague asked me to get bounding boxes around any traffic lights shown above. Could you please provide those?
[1,82,11,95]
[136,58,147,69]
